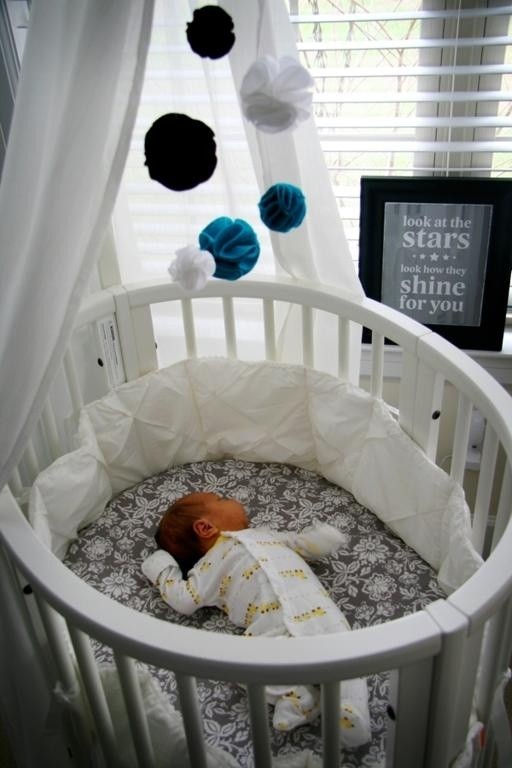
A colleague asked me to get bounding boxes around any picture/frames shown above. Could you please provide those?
[361,177,512,353]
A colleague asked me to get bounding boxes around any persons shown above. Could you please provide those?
[141,492,374,748]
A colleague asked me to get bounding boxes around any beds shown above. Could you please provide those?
[4,274,510,764]
